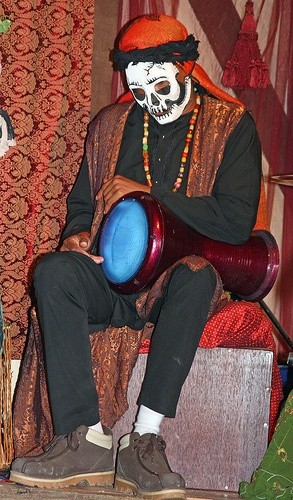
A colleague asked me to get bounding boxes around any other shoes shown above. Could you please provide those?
[8,423,115,489]
[113,432,188,499]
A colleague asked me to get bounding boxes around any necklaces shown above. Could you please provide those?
[142,96,202,191]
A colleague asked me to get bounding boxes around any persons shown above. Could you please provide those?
[11,14,260,500]
